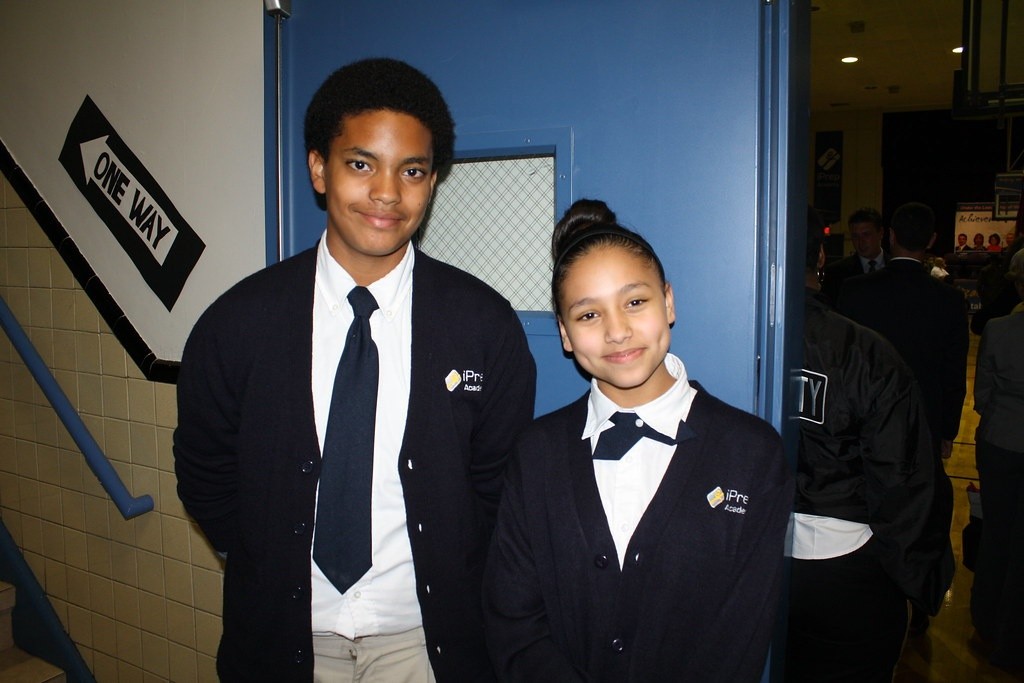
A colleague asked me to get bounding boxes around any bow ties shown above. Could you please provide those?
[592,411,699,460]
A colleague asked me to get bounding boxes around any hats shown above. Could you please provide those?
[1004,248,1024,281]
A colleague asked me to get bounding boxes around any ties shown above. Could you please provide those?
[867,260,877,273]
[312,286,379,593]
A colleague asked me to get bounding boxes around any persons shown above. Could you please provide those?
[784,203,957,683]
[482,196,796,683]
[977,253,1009,315]
[926,256,951,281]
[974,250,1024,665]
[825,207,890,299]
[956,232,1015,252]
[839,204,968,639]
[174,57,536,683]
